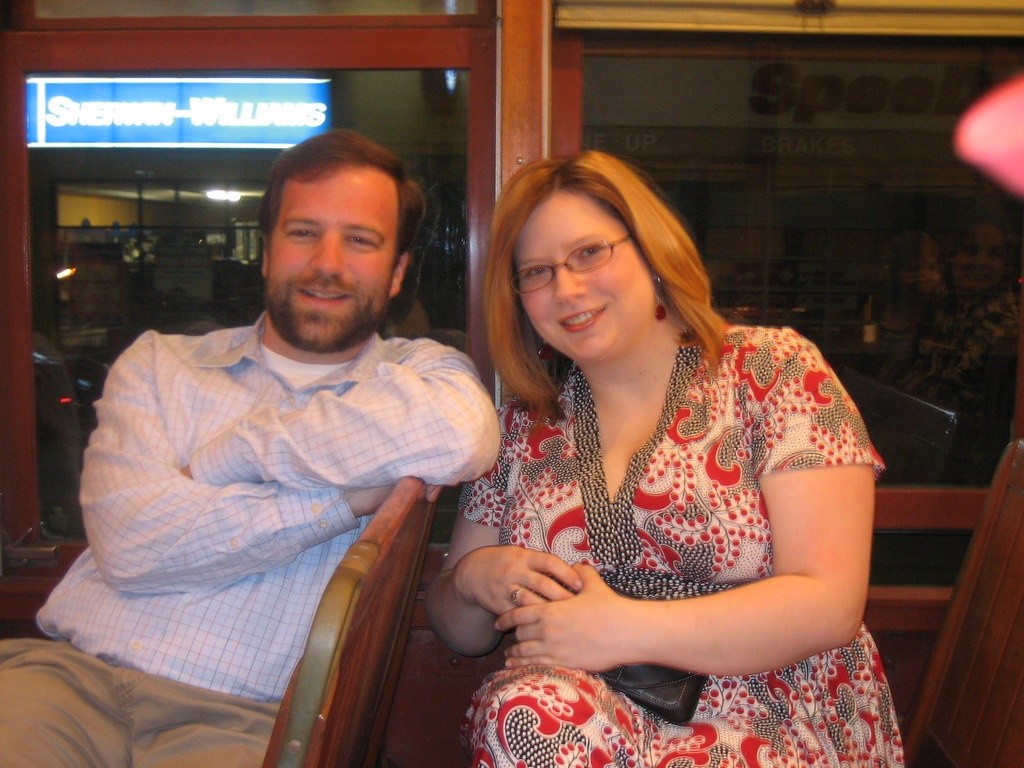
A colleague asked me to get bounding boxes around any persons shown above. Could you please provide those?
[0,130,499,768]
[426,151,906,768]
[832,209,1024,485]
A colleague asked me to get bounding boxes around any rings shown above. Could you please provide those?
[510,588,524,608]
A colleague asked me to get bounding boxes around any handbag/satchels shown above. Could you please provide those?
[583,584,709,726]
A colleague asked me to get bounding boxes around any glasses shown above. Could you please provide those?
[509,233,632,294]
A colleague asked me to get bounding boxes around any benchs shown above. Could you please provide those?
[0,426,1024,768]
